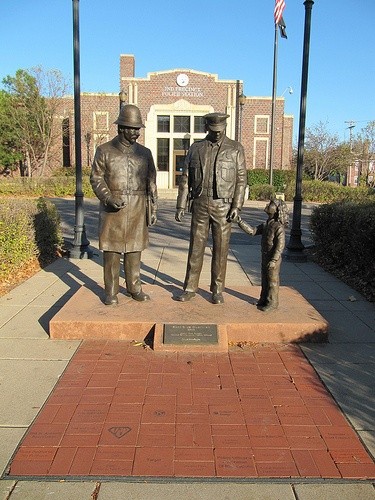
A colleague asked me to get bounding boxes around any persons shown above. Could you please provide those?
[175,113,247,305]
[89,105,158,306]
[236,199,289,312]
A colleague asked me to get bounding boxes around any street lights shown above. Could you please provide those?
[119,89,128,111]
[238,90,247,144]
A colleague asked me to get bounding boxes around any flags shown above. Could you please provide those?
[274,0,287,40]
